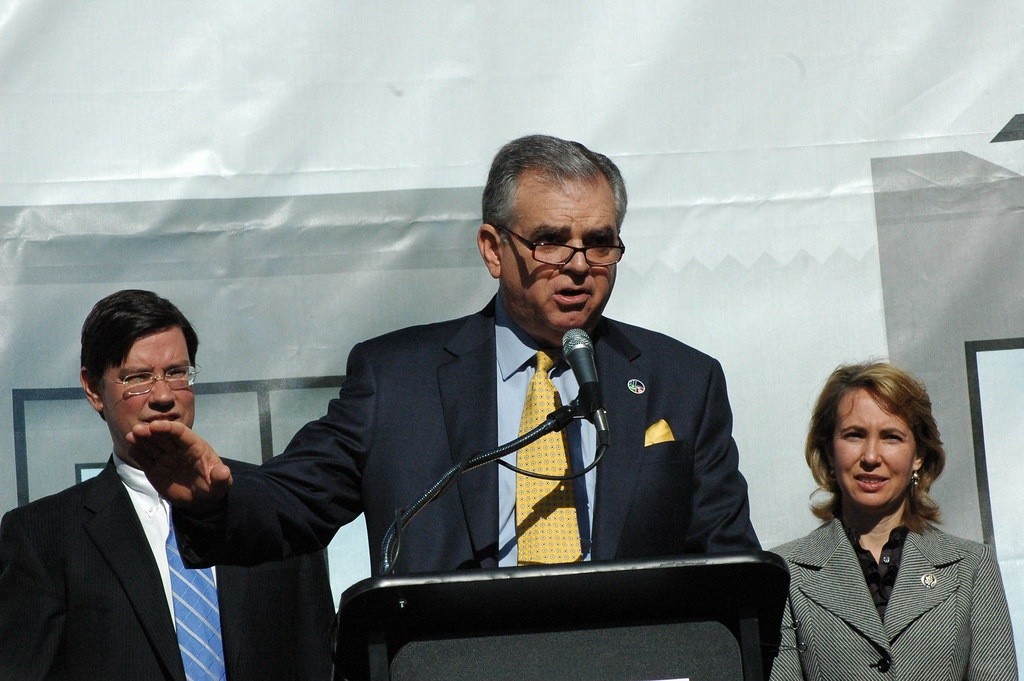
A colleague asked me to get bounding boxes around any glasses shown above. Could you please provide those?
[494,224,625,265]
[102,364,202,395]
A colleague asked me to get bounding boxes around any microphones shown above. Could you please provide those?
[562,329,611,447]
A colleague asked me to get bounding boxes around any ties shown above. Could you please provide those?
[514,349,584,564]
[166,506,229,681]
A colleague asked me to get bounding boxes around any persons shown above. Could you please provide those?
[765,355,1019,681]
[0,289,336,681]
[125,134,764,570]
